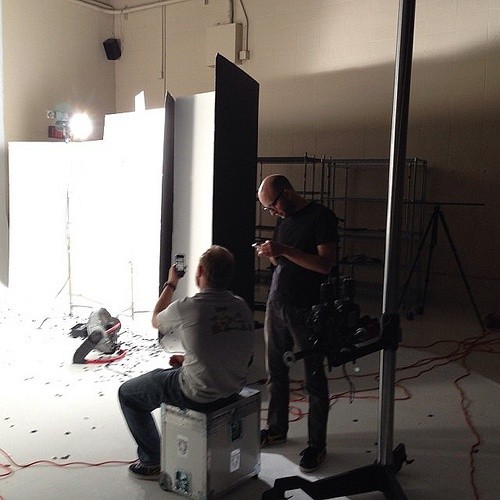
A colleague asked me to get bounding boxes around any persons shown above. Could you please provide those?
[119,245,255,481]
[258,174,337,473]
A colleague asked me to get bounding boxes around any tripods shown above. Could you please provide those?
[394,206,485,332]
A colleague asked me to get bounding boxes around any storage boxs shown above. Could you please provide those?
[159,386,261,500]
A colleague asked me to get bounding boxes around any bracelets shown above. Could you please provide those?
[163,282,176,291]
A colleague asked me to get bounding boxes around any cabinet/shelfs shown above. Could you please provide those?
[255,157,428,287]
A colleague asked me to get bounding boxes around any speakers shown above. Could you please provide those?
[103,38,121,60]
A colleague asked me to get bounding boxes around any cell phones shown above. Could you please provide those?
[176,254,184,272]
[252,242,263,248]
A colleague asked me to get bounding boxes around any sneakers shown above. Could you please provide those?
[299,441,326,472]
[260,427,287,448]
[128,462,160,479]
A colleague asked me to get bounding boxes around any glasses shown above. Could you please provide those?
[263,187,286,211]
